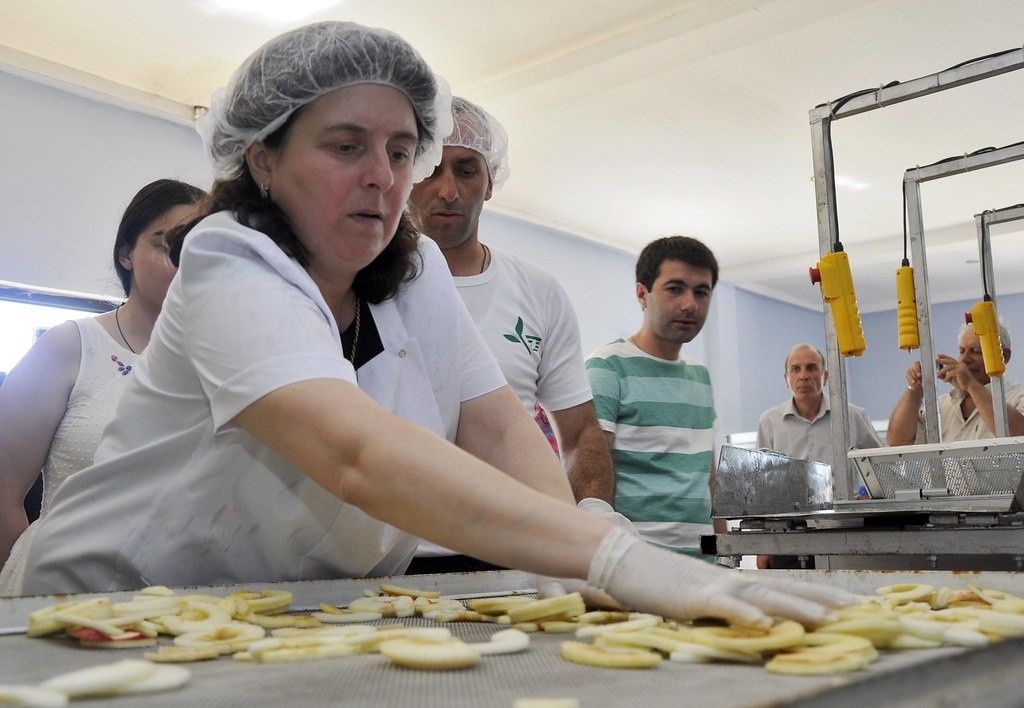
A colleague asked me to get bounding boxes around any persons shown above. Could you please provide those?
[883,319,1024,446]
[15,19,873,641]
[405,93,641,578]
[586,234,730,571]
[757,344,886,573]
[0,177,218,598]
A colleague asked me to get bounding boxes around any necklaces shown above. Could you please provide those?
[349,301,361,364]
[116,302,136,353]
[480,243,487,273]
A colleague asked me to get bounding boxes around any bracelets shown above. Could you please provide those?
[907,386,918,393]
